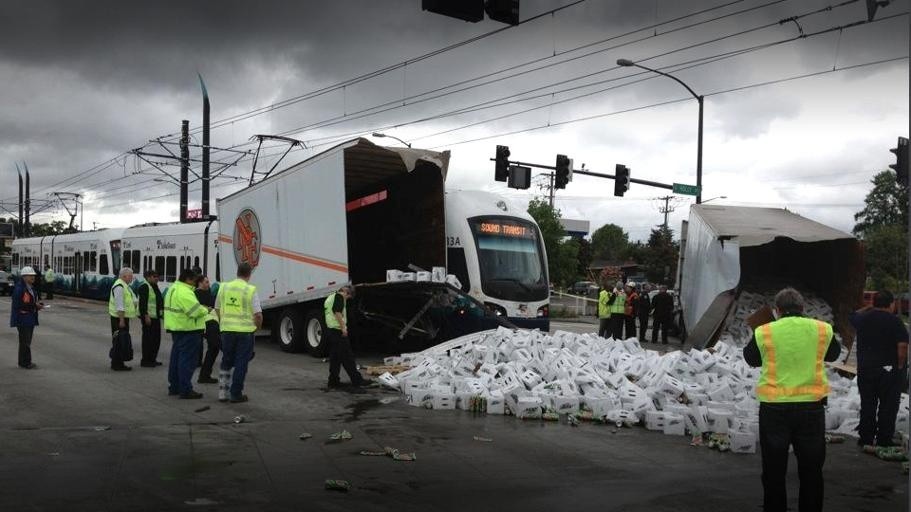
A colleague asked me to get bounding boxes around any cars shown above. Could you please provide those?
[0,270,16,295]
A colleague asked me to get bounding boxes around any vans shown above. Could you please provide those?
[574,281,597,295]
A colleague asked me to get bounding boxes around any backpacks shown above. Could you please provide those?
[109,327,134,361]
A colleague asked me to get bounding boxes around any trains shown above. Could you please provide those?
[9,187,552,333]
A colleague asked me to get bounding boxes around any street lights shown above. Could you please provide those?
[614,59,704,205]
[370,133,411,150]
[701,191,728,204]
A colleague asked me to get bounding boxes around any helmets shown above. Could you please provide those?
[21,265,36,277]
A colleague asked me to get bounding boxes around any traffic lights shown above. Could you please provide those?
[888,136,908,186]
[554,154,570,190]
[615,164,629,197]
[511,167,531,188]
[495,144,511,182]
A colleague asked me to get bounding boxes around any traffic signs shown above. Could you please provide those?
[673,182,700,197]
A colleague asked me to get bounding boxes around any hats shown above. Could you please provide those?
[625,281,636,288]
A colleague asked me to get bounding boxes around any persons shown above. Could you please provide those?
[598,281,674,344]
[44,264,55,299]
[10,266,44,369]
[324,285,375,389]
[744,287,840,510]
[214,261,263,403]
[107,266,255,397]
[32,265,43,302]
[851,289,909,447]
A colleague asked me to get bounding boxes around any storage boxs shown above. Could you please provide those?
[387,263,462,290]
[724,275,834,326]
[377,325,911,456]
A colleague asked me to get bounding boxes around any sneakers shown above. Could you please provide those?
[329,381,347,388]
[855,437,901,447]
[354,378,373,388]
[229,394,248,403]
[141,360,162,367]
[111,364,132,372]
[18,361,36,370]
[163,370,220,399]
